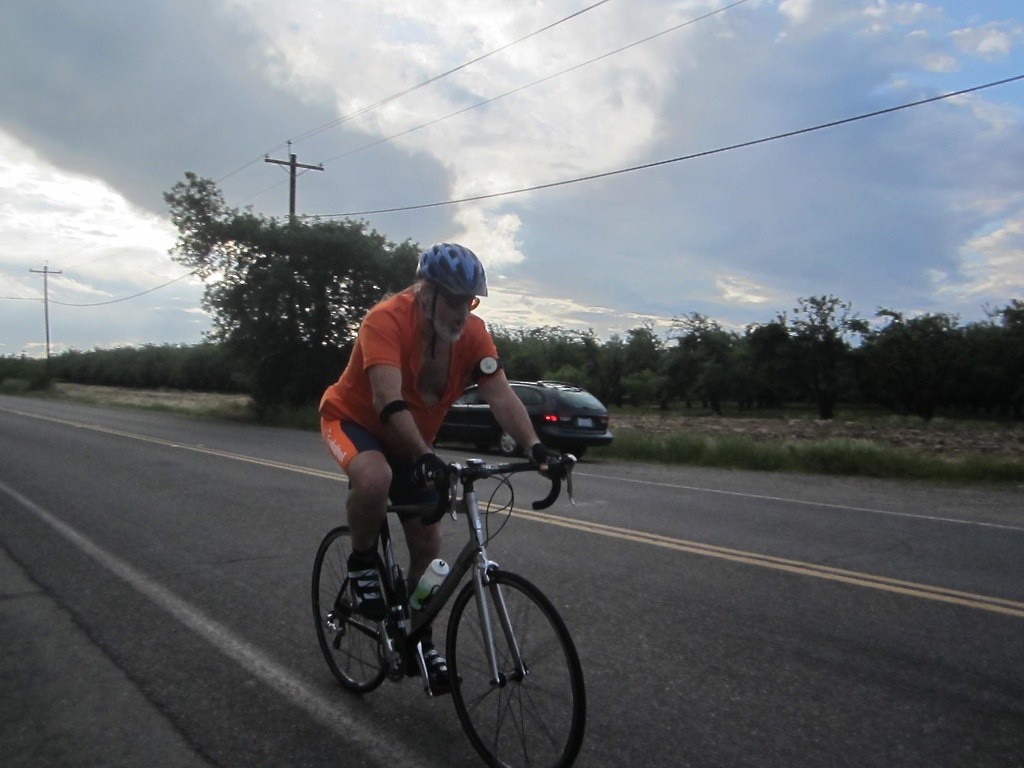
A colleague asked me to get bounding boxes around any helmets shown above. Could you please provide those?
[416,243,487,296]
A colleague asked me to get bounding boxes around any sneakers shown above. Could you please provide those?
[405,649,463,692]
[347,552,385,622]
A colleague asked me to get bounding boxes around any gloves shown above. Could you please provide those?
[409,453,451,495]
[527,444,570,481]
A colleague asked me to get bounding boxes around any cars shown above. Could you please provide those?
[434,382,613,459]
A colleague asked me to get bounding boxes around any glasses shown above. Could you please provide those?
[438,290,481,311]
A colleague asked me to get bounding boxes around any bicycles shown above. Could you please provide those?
[310,457,589,768]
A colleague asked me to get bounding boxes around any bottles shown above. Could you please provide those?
[409,558,450,610]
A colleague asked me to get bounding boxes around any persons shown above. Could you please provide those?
[318,244,562,696]
